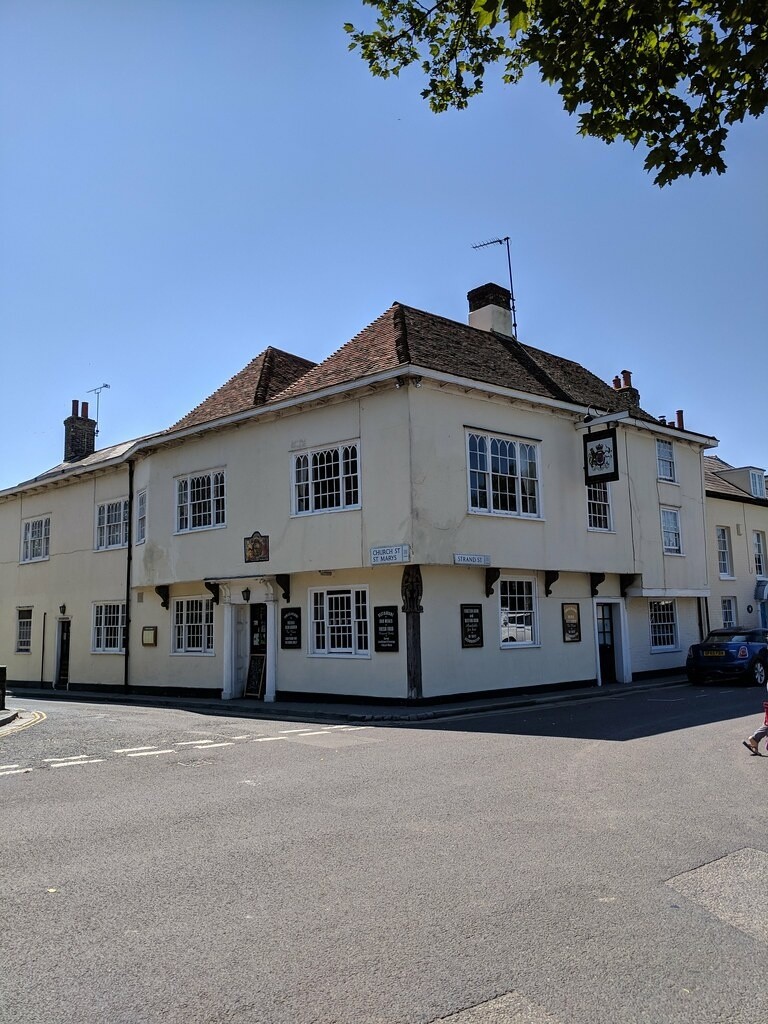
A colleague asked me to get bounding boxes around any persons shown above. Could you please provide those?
[742,679,768,756]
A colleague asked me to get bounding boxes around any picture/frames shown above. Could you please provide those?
[561,602,582,642]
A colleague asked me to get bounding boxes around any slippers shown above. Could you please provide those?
[743,741,761,756]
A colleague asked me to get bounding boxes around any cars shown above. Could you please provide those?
[686,626,768,687]
